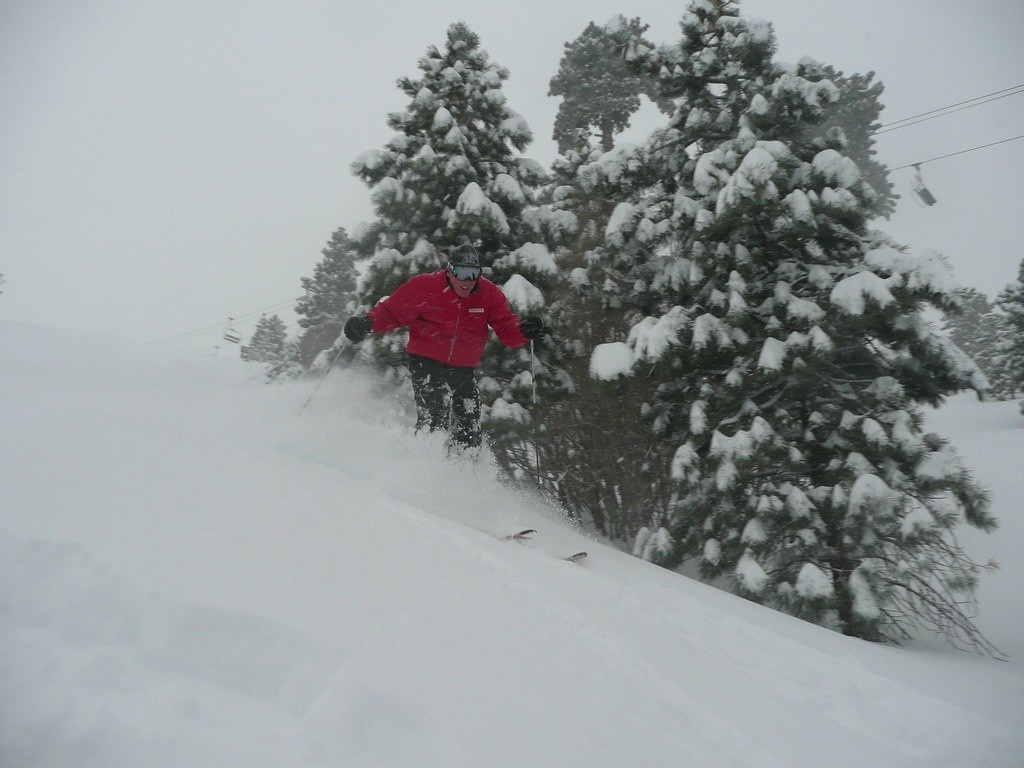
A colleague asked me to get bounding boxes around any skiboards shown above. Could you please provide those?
[466,528,589,563]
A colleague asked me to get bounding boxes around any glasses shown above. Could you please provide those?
[452,265,483,281]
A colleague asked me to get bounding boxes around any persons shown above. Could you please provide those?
[344,245,545,449]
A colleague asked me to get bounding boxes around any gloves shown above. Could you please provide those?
[344,315,372,344]
[518,315,544,338]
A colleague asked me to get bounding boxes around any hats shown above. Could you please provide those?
[449,243,479,266]
